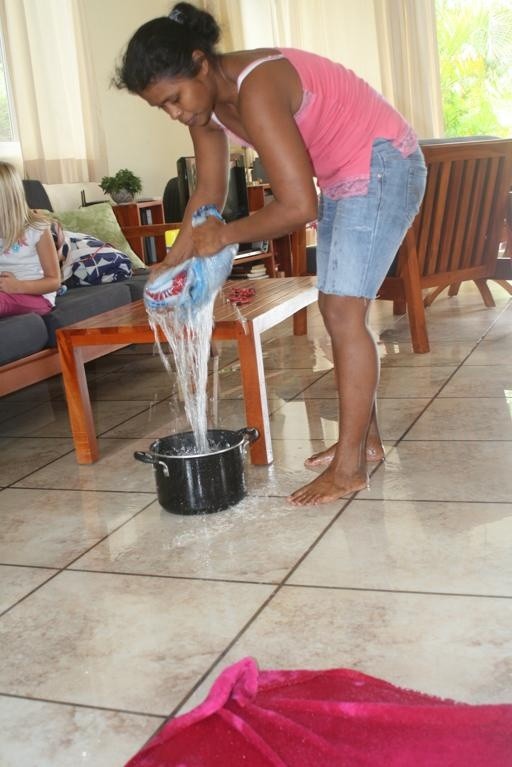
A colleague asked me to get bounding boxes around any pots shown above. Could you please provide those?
[134,426,261,514]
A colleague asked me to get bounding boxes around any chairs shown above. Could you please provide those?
[292,135,512,354]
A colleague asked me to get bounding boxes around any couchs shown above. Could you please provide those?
[0,181,189,398]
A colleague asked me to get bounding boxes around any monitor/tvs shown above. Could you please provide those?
[163,153,249,223]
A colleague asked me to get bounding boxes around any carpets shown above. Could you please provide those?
[123,656,511,767]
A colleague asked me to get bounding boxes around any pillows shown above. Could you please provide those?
[44,202,146,270]
[60,231,133,286]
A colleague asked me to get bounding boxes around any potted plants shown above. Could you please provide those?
[98,168,143,203]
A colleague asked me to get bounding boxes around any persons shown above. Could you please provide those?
[106,1,429,508]
[0,161,62,321]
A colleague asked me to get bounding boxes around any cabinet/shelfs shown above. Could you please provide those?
[108,184,291,277]
